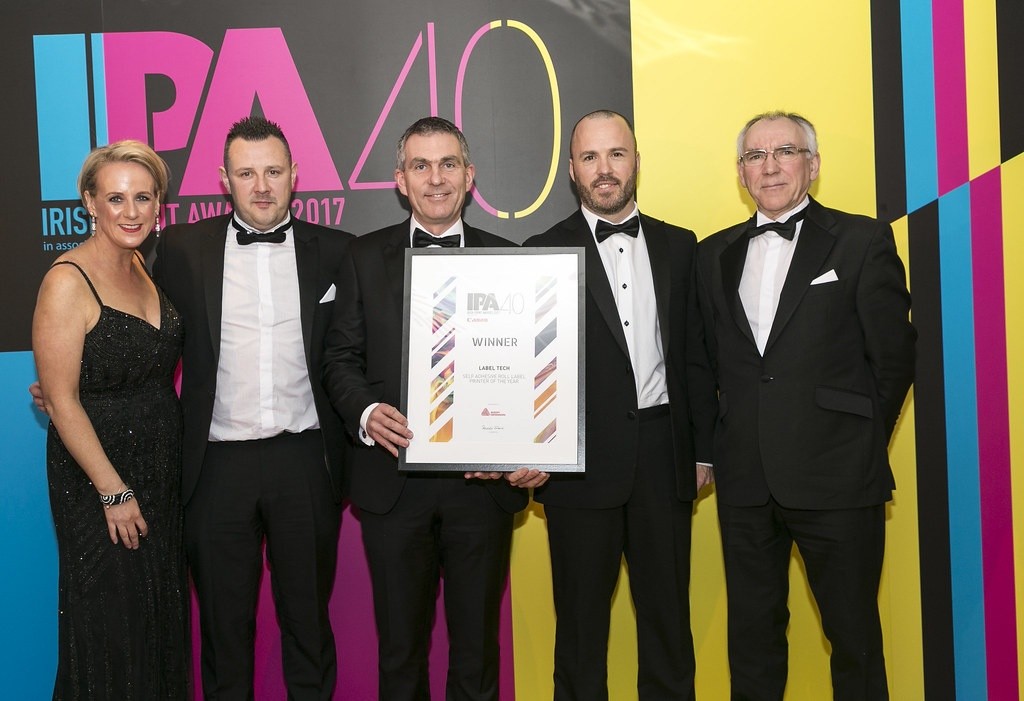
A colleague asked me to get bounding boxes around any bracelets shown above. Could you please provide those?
[98,486,135,509]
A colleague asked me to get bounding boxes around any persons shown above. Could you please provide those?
[503,109,720,701]
[29,117,355,701]
[31,138,194,701]
[696,110,921,701]
[321,118,529,701]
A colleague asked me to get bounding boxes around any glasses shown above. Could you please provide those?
[739,145,811,167]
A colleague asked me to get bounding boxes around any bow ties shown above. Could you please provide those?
[412,228,461,249]
[231,216,292,245]
[747,203,810,241]
[595,215,639,244]
